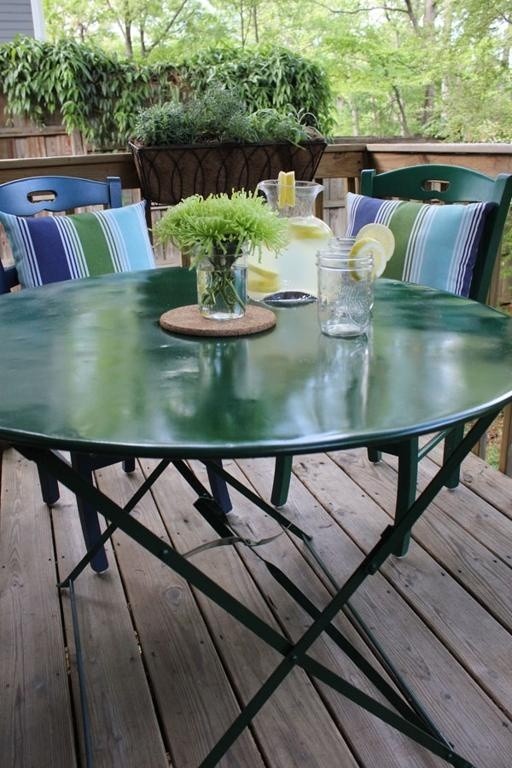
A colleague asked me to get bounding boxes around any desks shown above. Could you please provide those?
[1,265,512,766]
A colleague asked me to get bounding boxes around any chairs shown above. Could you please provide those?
[267,162,512,559]
[0,173,235,573]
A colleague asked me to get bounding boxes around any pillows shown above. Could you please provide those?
[0,198,161,291]
[341,189,499,302]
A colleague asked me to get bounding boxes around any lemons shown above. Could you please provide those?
[353,222,396,261]
[246,261,282,295]
[286,222,331,241]
[277,169,296,210]
[350,237,388,281]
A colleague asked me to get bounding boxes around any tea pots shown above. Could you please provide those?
[248,178,342,306]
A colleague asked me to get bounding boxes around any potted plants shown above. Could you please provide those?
[124,83,331,203]
[149,186,290,321]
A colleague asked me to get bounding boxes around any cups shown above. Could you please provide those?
[315,235,379,337]
